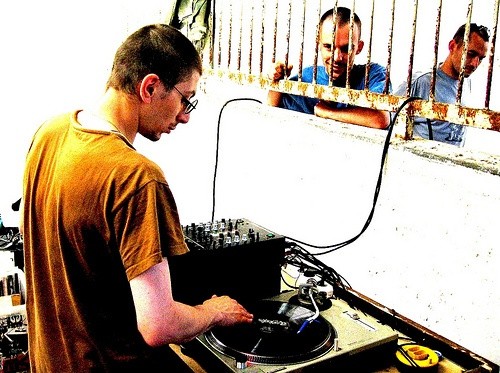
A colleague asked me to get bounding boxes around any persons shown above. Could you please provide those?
[393,23,491,148]
[18,23,254,373]
[267,7,394,130]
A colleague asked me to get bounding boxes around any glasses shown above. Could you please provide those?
[168,81,198,114]
